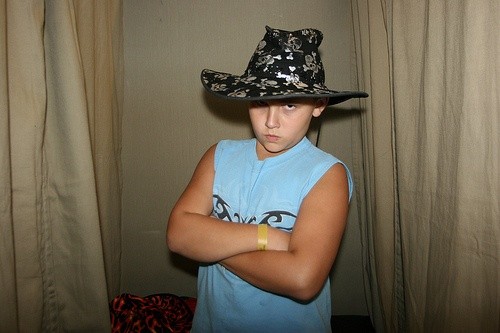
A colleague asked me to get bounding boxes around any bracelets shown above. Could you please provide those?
[257,223,267,252]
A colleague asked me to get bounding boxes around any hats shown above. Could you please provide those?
[201,25,369,105]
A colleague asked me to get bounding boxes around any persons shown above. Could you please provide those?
[166,26,353,333]
